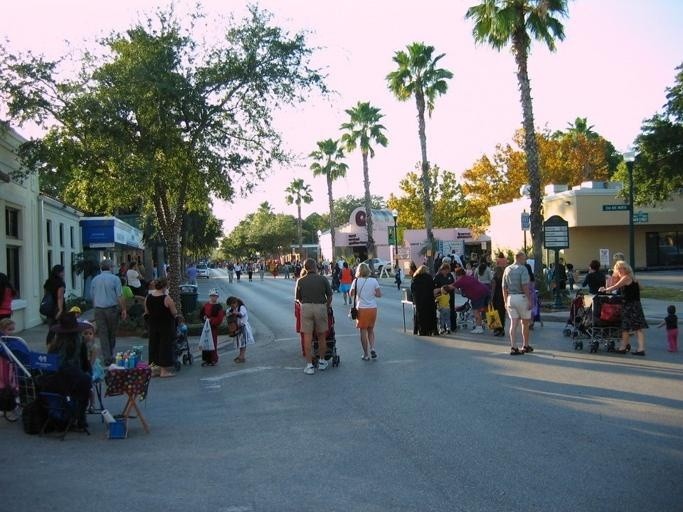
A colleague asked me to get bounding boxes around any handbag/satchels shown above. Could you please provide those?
[597,300,622,323]
[198,318,215,351]
[39,291,56,315]
[22,400,50,434]
[485,302,503,330]
[351,277,358,320]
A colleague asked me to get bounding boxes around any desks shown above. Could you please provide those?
[108,361,151,433]
[401,300,445,335]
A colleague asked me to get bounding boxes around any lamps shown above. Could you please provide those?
[563,199,570,206]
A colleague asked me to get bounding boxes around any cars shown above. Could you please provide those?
[355,255,389,272]
[196,262,210,279]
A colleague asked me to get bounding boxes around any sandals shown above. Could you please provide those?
[88,404,95,415]
[511,347,525,355]
[522,345,533,352]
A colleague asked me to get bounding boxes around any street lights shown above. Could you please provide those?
[620,140,638,283]
[316,230,322,272]
[391,207,401,286]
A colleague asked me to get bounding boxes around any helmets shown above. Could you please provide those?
[209,288,219,297]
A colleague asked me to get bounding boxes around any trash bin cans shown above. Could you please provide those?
[180,285,200,316]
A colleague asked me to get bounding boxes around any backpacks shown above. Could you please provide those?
[226,304,247,337]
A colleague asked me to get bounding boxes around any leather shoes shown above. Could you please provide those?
[159,372,175,378]
[234,358,244,362]
[150,371,160,377]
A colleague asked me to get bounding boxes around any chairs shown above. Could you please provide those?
[41,378,103,437]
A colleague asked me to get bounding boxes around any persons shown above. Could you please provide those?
[347,261,381,361]
[116,260,199,291]
[42,264,65,347]
[223,297,249,364]
[393,265,401,290]
[198,287,218,366]
[144,275,180,379]
[526,253,605,327]
[0,318,14,337]
[226,258,302,282]
[598,260,647,355]
[317,259,358,304]
[501,251,534,354]
[410,251,514,336]
[608,253,626,285]
[43,304,105,432]
[655,304,678,352]
[0,271,15,317]
[295,256,332,374]
[88,258,126,360]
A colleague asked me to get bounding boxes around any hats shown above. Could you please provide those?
[68,306,82,313]
[49,313,92,333]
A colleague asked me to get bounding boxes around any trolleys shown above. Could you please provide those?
[0,335,54,424]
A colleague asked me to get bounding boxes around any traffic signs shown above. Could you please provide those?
[601,203,629,212]
[630,213,647,222]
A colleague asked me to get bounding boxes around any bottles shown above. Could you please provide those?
[100,409,115,423]
[115,349,138,369]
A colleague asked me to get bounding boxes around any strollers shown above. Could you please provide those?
[456,291,474,321]
[172,315,195,370]
[562,290,624,353]
[309,307,341,369]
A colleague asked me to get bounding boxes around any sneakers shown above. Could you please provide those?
[361,354,370,361]
[14,405,23,417]
[630,350,645,356]
[370,348,377,358]
[5,409,19,422]
[303,366,314,374]
[616,343,631,353]
[470,328,484,334]
[319,360,328,370]
